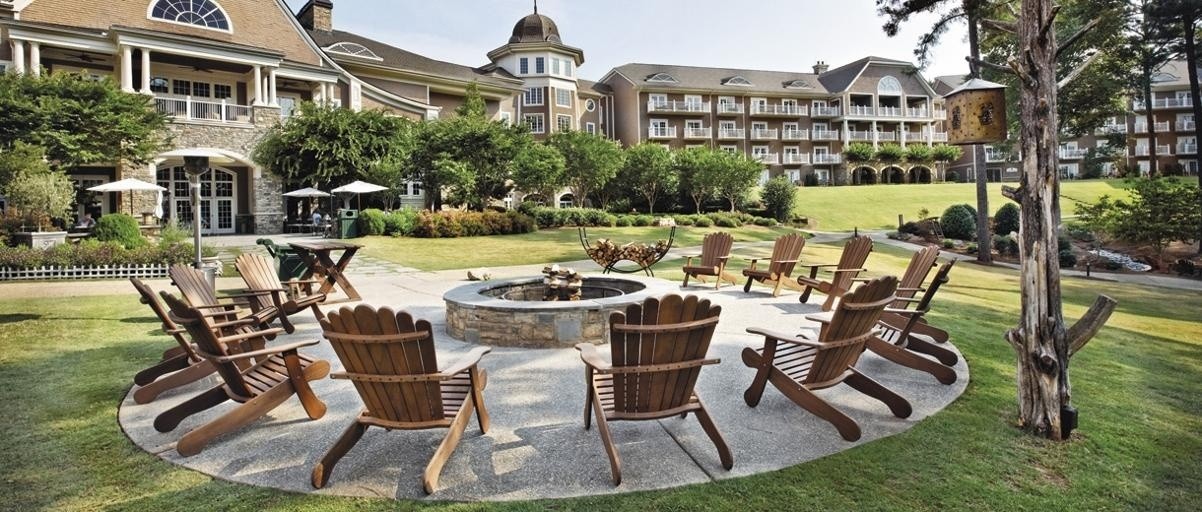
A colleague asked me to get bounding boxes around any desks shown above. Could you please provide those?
[287,240,364,303]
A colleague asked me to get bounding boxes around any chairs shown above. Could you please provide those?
[846,244,943,343]
[682,232,736,290]
[310,303,492,495]
[807,256,958,390]
[151,289,328,460]
[169,260,281,356]
[745,273,915,441]
[744,234,809,301]
[288,218,333,236]
[797,235,877,314]
[235,254,328,336]
[129,273,273,405]
[574,294,734,485]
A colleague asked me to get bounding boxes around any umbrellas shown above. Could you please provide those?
[330,179,390,210]
[281,187,337,217]
[85,177,168,218]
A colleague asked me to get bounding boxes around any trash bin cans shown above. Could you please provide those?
[257,238,314,283]
[337,209,359,239]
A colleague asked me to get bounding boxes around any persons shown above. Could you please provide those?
[76,213,97,228]
[322,211,332,226]
[313,208,321,236]
[321,220,334,239]
